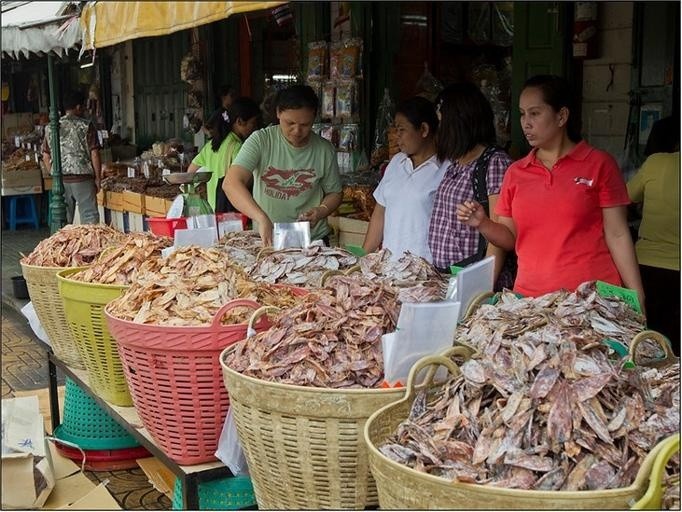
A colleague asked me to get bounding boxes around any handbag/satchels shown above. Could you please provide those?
[215,140,253,225]
[447,145,518,292]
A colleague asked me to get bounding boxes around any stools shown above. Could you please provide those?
[4,196,39,231]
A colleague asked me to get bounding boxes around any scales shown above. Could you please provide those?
[159,171,213,219]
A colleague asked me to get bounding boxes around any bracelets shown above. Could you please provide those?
[318,202,330,211]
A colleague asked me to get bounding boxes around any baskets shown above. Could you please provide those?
[220,306,471,510]
[54,246,134,407]
[362,356,681,510]
[18,255,89,370]
[106,283,312,468]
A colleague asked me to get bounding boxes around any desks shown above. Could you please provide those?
[47,349,260,510]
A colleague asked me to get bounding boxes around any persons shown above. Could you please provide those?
[222,86,341,246]
[456,75,646,321]
[41,90,103,223]
[360,98,453,264]
[428,86,518,294]
[622,150,678,352]
[186,99,259,218]
